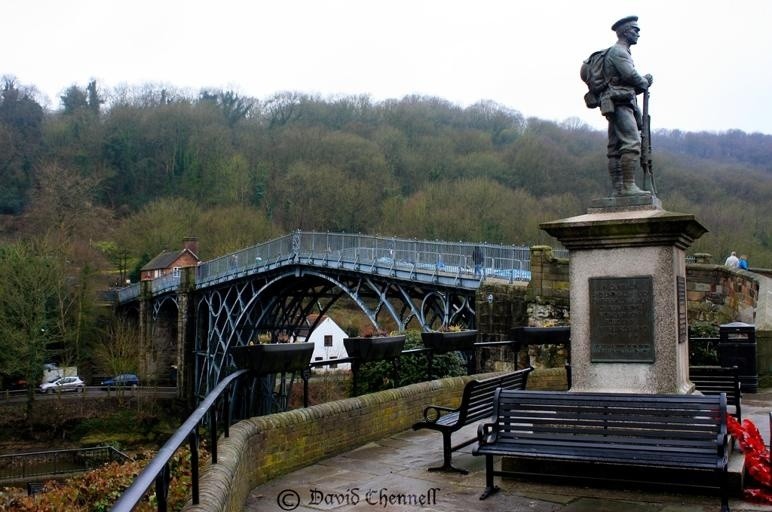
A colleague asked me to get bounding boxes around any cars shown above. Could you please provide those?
[101,374,139,391]
[39,377,86,394]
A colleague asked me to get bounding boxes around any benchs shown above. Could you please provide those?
[412,366,741,512]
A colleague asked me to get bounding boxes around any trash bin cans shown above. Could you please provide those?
[719,321,759,394]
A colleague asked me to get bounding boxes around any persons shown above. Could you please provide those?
[597,13,654,198]
[472,246,484,276]
[737,254,749,270]
[724,251,739,269]
